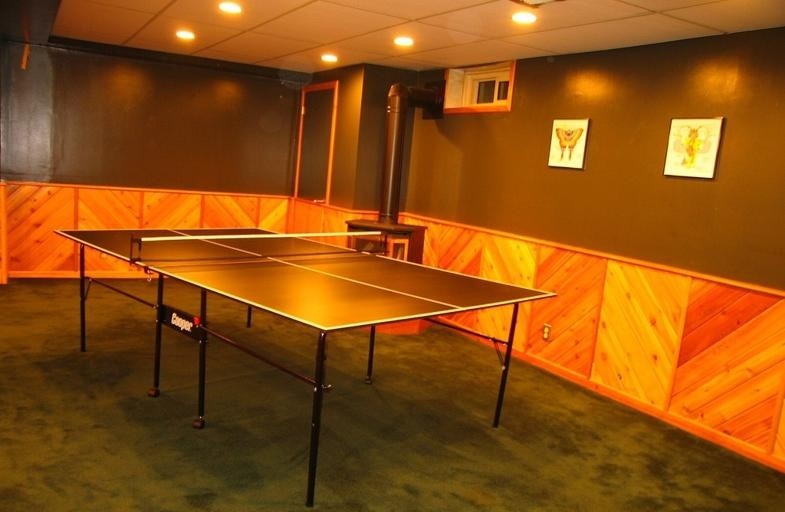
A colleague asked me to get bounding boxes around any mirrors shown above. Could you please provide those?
[296,80,339,206]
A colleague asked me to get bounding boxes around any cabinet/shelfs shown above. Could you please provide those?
[346,220,428,266]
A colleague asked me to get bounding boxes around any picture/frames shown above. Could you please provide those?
[548,119,590,171]
[663,117,724,180]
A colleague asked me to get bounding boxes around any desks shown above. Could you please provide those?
[55,228,557,507]
